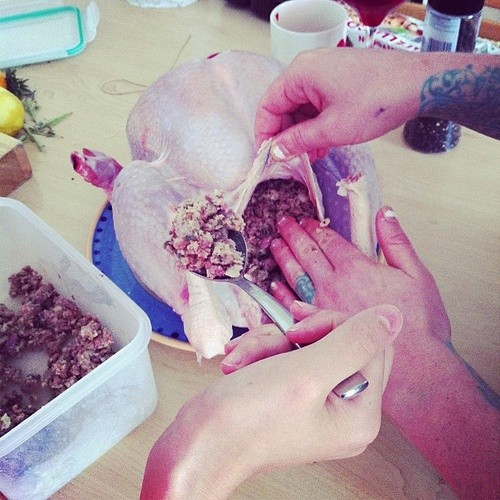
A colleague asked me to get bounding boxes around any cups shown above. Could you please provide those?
[270,0,348,65]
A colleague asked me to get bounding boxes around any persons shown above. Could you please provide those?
[138,46,500,500]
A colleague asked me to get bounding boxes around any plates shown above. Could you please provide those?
[91,145,384,356]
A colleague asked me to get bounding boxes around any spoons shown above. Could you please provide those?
[171,220,368,400]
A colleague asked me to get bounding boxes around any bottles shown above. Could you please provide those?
[402,0,485,153]
